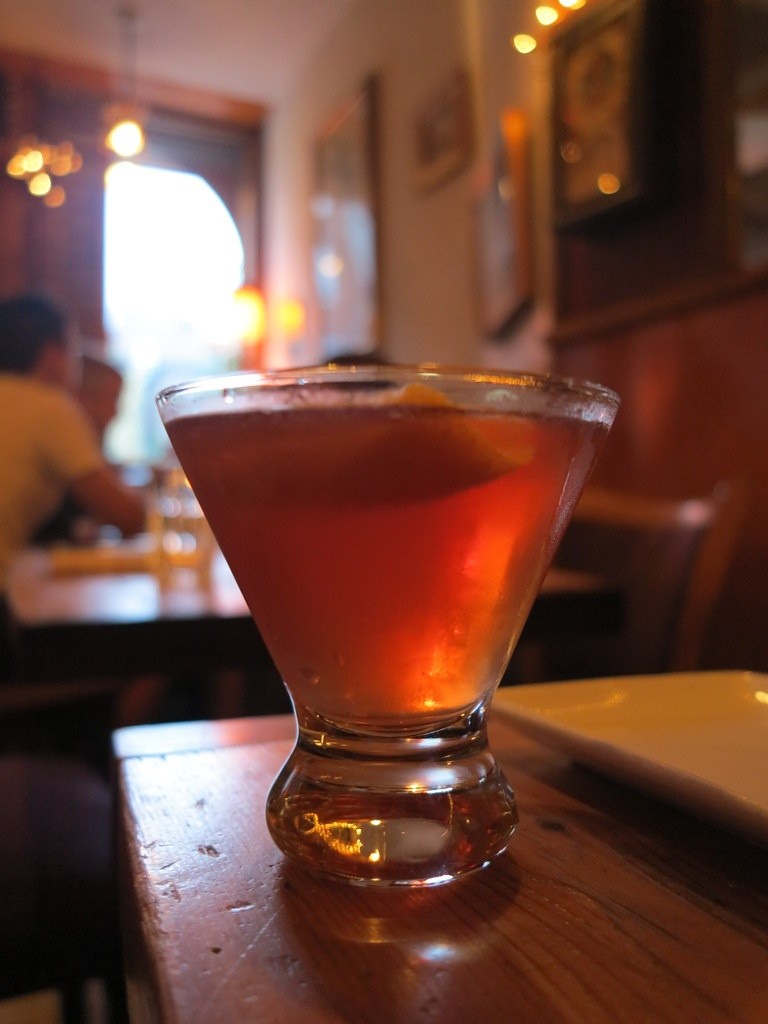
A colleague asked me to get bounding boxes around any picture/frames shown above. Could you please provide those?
[401,68,478,197]
[468,128,533,340]
[299,76,381,363]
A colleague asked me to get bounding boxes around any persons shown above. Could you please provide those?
[0,295,160,770]
[205,352,392,719]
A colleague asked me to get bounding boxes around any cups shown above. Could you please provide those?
[153,365,619,890]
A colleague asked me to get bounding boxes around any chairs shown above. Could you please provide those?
[522,472,755,692]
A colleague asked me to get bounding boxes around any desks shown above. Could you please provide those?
[58,671,768,1023]
[13,532,625,1023]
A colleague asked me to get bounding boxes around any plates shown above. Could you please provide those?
[486,669,768,835]
